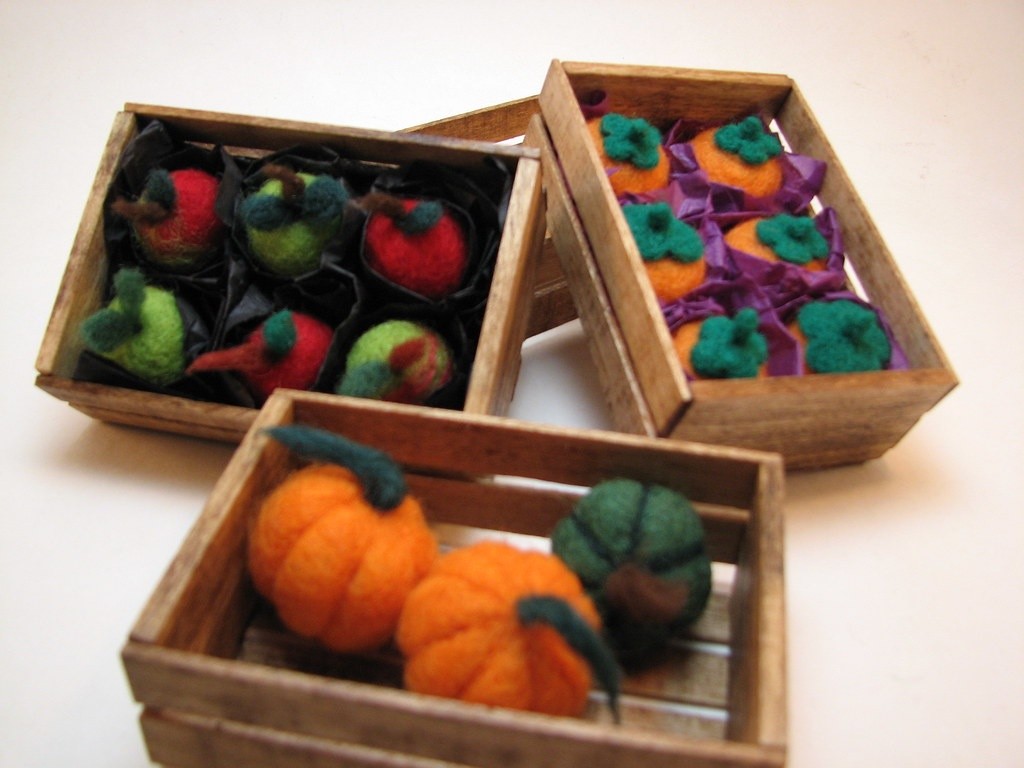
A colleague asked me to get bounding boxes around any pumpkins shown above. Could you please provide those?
[245,422,713,719]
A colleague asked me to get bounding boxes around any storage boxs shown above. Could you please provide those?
[524,59,961,474]
[388,94,576,360]
[33,101,546,443]
[120,388,787,768]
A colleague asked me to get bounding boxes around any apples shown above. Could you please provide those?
[74,169,466,402]
